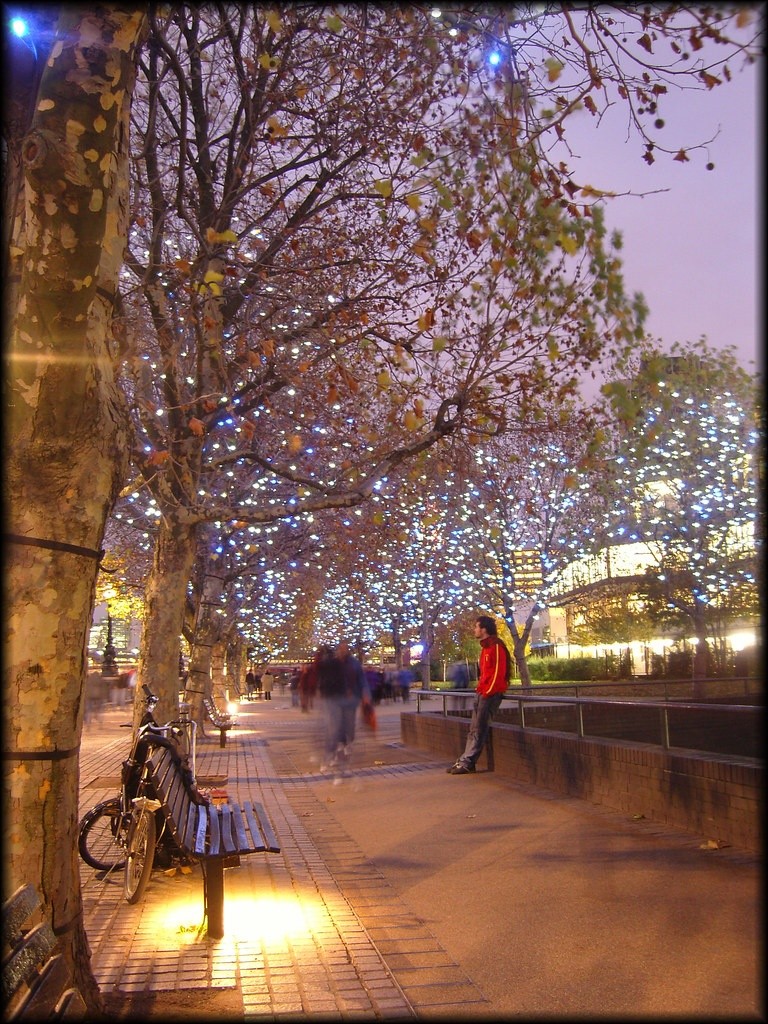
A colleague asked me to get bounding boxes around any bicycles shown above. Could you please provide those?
[78,683,195,904]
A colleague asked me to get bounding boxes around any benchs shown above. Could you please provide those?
[202,698,232,748]
[210,695,239,719]
[141,735,280,939]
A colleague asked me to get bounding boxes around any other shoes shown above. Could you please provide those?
[446,763,476,774]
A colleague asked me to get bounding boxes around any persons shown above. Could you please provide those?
[84,668,139,734]
[446,615,510,775]
[242,639,471,773]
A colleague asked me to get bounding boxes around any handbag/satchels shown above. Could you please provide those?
[362,703,376,728]
